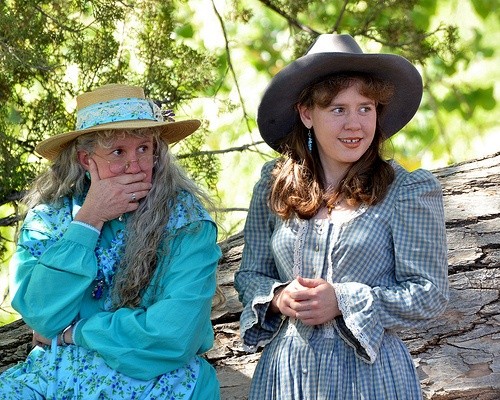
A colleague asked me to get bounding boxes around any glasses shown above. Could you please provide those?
[91,152,158,174]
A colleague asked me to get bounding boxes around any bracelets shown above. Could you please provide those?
[60,321,77,347]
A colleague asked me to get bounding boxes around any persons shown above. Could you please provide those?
[0,83,224,399]
[232,33,449,400]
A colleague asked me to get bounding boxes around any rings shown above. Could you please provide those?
[130,192,136,201]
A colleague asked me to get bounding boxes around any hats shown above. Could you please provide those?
[256,34,423,155]
[35,84,202,164]
